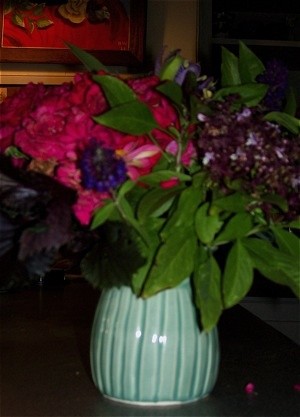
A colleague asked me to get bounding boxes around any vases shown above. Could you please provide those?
[91,242,219,405]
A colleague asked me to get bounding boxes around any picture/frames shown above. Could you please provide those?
[0,0,148,67]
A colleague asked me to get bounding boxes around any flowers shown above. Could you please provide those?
[1,38,300,332]
